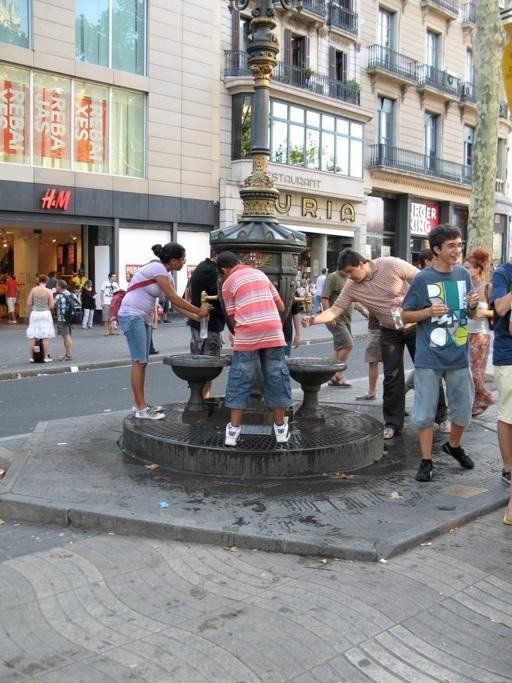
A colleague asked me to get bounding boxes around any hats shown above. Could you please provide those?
[86,280,93,286]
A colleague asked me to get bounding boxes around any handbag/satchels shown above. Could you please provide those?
[108,260,166,317]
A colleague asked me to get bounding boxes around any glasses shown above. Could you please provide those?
[180,259,187,264]
[441,244,465,249]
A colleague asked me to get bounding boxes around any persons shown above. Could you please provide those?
[399,224,481,482]
[320,267,369,388]
[0,279,8,318]
[72,269,87,299]
[125,273,133,285]
[158,275,176,324]
[504,493,512,525]
[460,248,498,418]
[55,271,62,280]
[54,280,83,361]
[117,241,212,421]
[45,271,58,320]
[12,275,20,319]
[80,279,97,329]
[100,272,119,336]
[190,251,226,401]
[151,297,159,329]
[5,272,17,324]
[182,269,194,322]
[311,268,327,317]
[300,245,451,440]
[300,272,307,287]
[213,251,292,447]
[403,249,437,417]
[292,282,306,312]
[310,282,324,317]
[489,258,511,483]
[26,274,54,363]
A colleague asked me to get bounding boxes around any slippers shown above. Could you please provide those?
[503,507,512,525]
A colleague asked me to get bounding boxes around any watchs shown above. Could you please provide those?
[467,303,477,310]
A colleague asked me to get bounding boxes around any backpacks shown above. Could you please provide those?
[56,292,83,330]
[108,279,157,316]
[33,339,51,363]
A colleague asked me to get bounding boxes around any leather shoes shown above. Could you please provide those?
[29,359,35,364]
[44,358,53,363]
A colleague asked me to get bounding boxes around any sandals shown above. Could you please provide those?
[333,375,352,386]
[328,379,336,387]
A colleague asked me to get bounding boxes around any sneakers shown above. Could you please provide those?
[225,422,241,447]
[355,392,376,401]
[58,355,67,361]
[135,406,166,420]
[442,442,475,469]
[63,356,73,362]
[502,469,511,484]
[384,427,395,439]
[273,416,291,444]
[415,459,433,481]
[8,320,17,325]
[439,420,451,433]
[132,404,163,413]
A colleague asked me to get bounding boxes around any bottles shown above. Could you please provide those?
[391,304,403,329]
[200,316,208,339]
